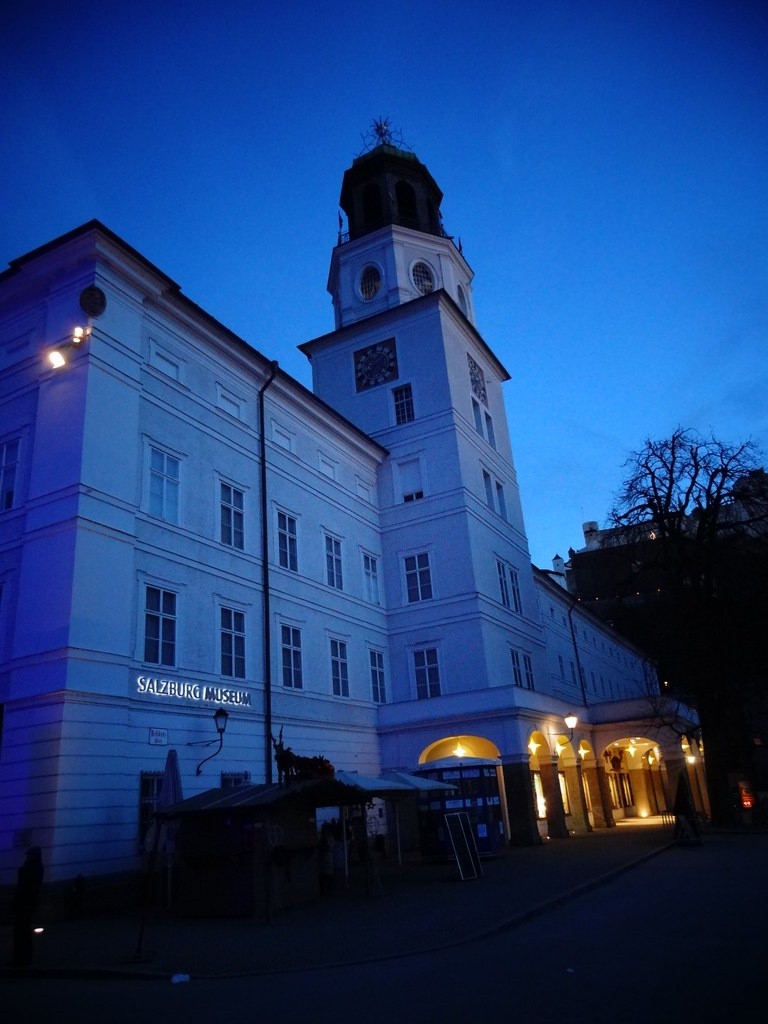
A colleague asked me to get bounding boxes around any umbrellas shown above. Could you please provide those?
[276,770,458,890]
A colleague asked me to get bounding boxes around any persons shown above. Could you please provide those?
[318,826,337,898]
[6,845,44,967]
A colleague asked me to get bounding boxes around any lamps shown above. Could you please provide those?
[48,347,68,368]
[72,326,90,347]
[546,711,578,755]
[188,708,228,774]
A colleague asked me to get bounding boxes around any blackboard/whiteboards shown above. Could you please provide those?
[444,811,484,882]
